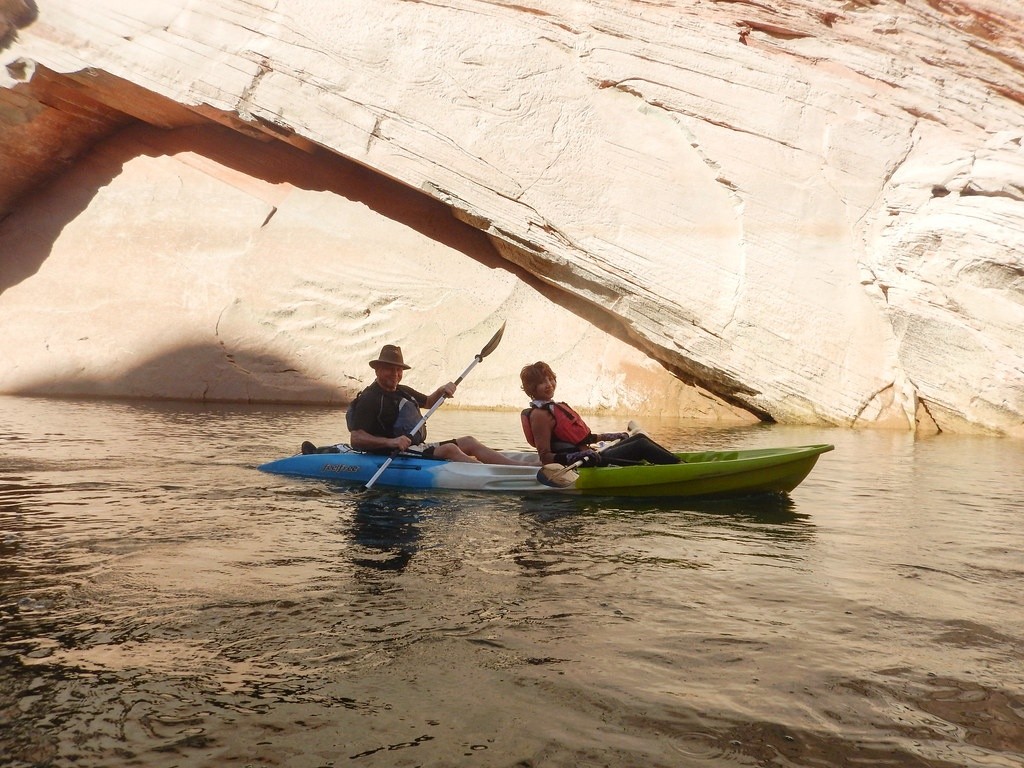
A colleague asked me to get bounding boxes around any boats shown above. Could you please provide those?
[257,443,836,503]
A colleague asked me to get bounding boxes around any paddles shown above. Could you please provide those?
[536,419,649,489]
[359,319,507,492]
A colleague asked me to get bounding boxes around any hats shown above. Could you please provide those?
[369,344,412,369]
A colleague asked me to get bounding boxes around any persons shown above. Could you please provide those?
[346,345,542,467]
[519,361,717,467]
